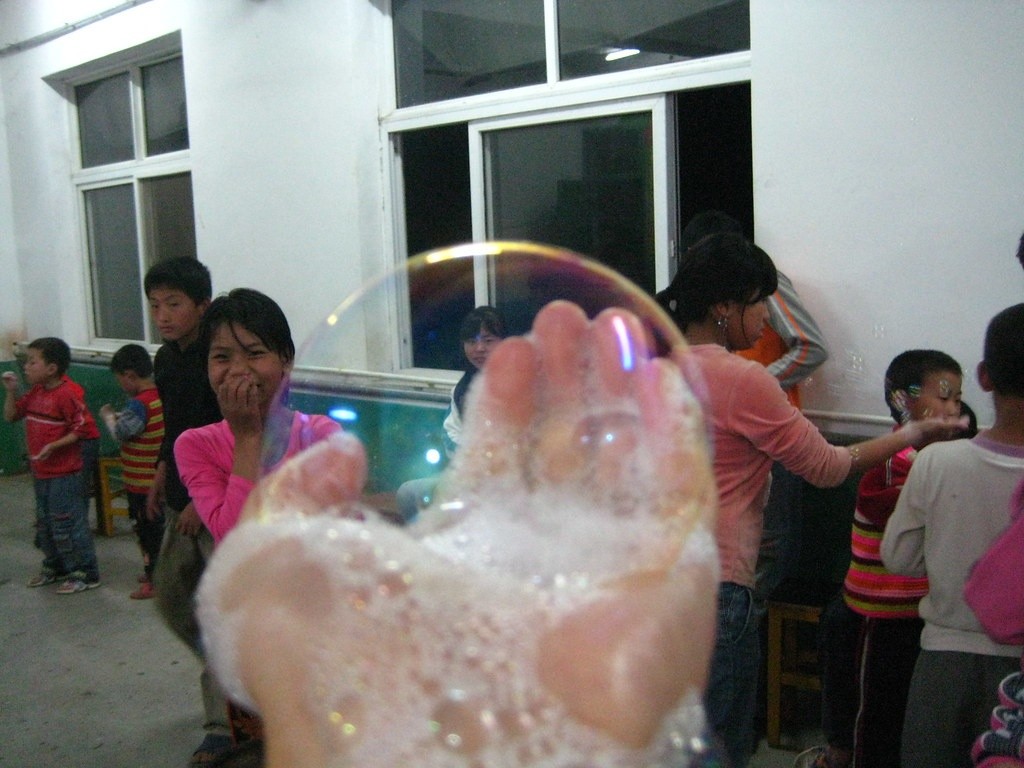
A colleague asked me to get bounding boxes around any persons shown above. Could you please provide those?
[140,254,265,767]
[394,306,510,521]
[221,302,718,768]
[98,343,166,598]
[964,477,1024,768]
[1,337,101,594]
[171,287,340,544]
[876,303,1024,768]
[685,211,829,752]
[647,234,970,768]
[810,349,963,768]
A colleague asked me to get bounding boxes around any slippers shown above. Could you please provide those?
[187,733,231,768]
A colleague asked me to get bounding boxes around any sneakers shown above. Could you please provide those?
[27,567,68,587]
[56,570,102,594]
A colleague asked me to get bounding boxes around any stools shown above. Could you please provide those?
[98,456,129,535]
[763,582,833,748]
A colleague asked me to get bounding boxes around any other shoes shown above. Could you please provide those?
[130,576,155,599]
